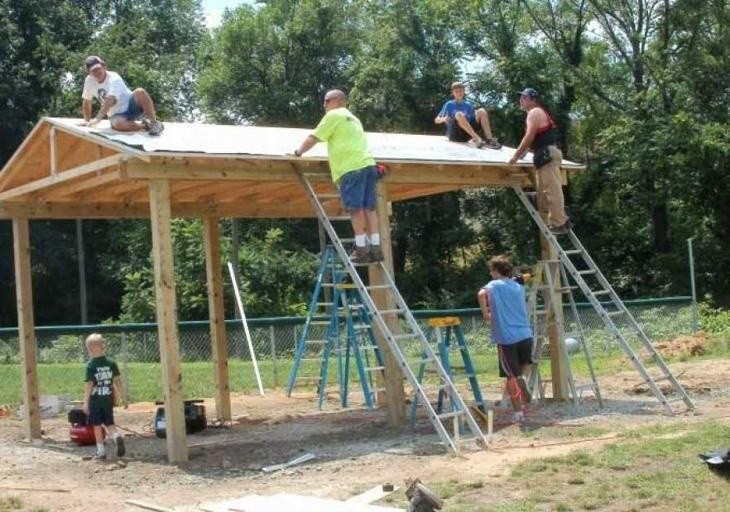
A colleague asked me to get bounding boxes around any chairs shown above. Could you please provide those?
[350,245,385,263]
[512,411,525,424]
[475,136,502,150]
[142,114,165,135]
[516,375,533,404]
[92,453,106,461]
[547,218,572,234]
[114,433,125,457]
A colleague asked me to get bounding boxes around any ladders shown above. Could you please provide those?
[500,169,697,413]
[284,162,490,459]
[286,245,385,410]
[500,259,605,412]
[407,316,485,433]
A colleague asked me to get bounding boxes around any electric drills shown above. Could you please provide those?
[512,273,534,284]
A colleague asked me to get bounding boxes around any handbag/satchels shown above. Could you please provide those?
[293,149,302,157]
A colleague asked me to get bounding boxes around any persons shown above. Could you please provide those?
[76,54,164,135]
[508,88,574,235]
[84,333,129,461]
[433,81,503,149]
[286,90,386,262]
[477,255,537,424]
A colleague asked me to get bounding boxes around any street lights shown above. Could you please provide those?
[534,147,553,170]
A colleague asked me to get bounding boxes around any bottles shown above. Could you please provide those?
[516,88,539,97]
[85,55,106,72]
